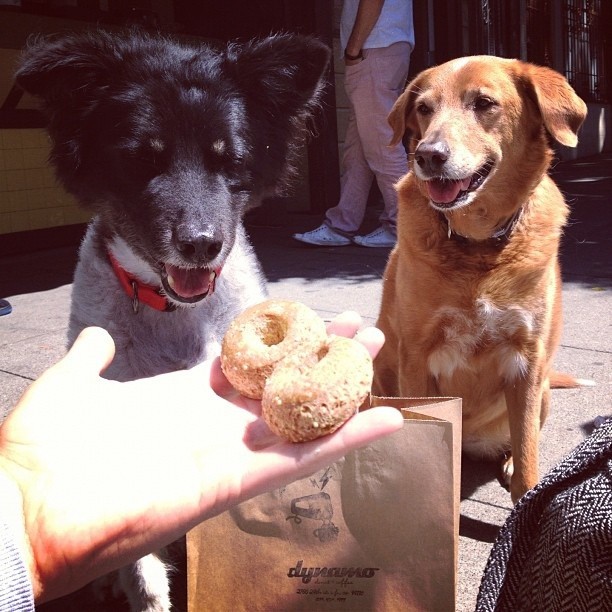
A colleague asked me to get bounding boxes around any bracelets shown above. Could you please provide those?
[344,49,362,60]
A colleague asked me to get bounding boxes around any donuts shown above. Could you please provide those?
[221,299,326,400]
[261,335,373,443]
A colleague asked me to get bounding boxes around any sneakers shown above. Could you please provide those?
[291,222,352,246]
[351,225,397,248]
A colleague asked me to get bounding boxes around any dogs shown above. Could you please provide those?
[0,21,334,612]
[370,54,597,510]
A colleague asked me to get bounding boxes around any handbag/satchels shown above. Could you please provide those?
[185,391,463,612]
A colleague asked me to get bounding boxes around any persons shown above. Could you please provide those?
[292,0,417,249]
[1,307,403,611]
